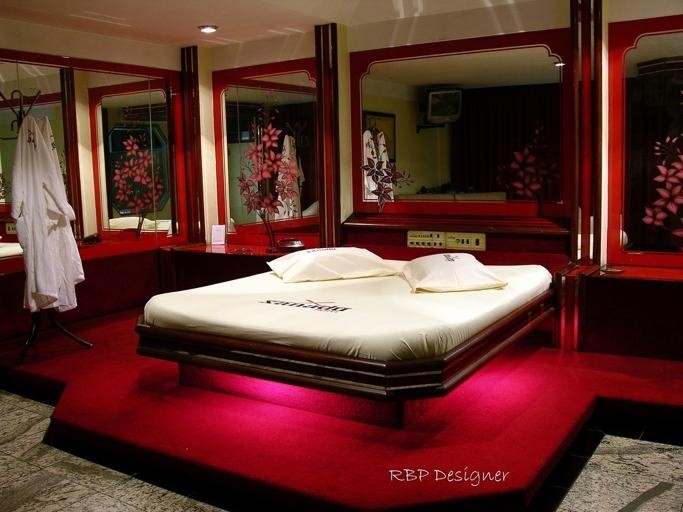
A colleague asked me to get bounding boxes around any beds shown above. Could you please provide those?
[136,259,553,430]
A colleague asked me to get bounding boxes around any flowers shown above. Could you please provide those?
[509,146,548,196]
[643,136,682,237]
[362,132,414,214]
[236,113,307,248]
[112,131,164,241]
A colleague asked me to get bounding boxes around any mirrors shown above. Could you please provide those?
[1,92,71,210]
[349,27,581,221]
[1,52,204,270]
[607,14,683,268]
[212,56,318,246]
[86,81,179,241]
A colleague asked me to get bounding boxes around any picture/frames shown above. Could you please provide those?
[363,110,397,164]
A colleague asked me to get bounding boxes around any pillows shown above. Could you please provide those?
[266,248,395,281]
[400,250,508,292]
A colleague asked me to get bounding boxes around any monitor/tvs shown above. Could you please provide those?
[424,88,462,124]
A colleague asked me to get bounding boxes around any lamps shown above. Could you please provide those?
[198,26,218,33]
